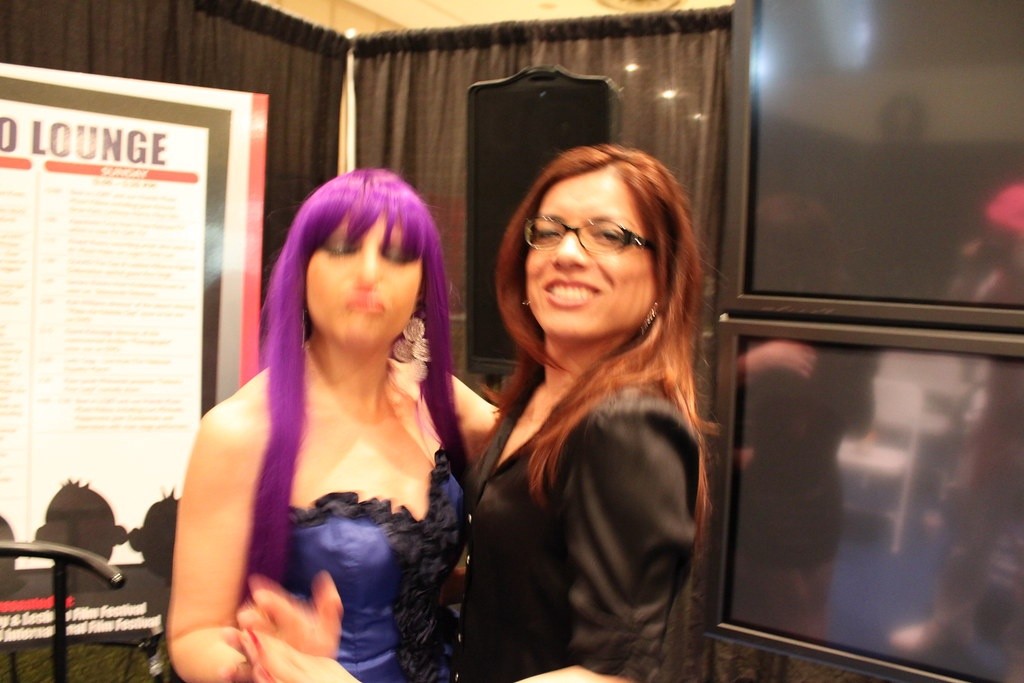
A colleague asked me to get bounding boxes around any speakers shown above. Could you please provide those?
[464,65,624,378]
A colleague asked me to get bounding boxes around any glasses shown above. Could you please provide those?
[519,214,654,261]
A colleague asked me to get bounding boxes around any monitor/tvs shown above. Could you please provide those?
[704,0,1024,683]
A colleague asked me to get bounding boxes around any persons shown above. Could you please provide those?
[241,145,722,683]
[168,168,502,683]
[731,341,875,640]
